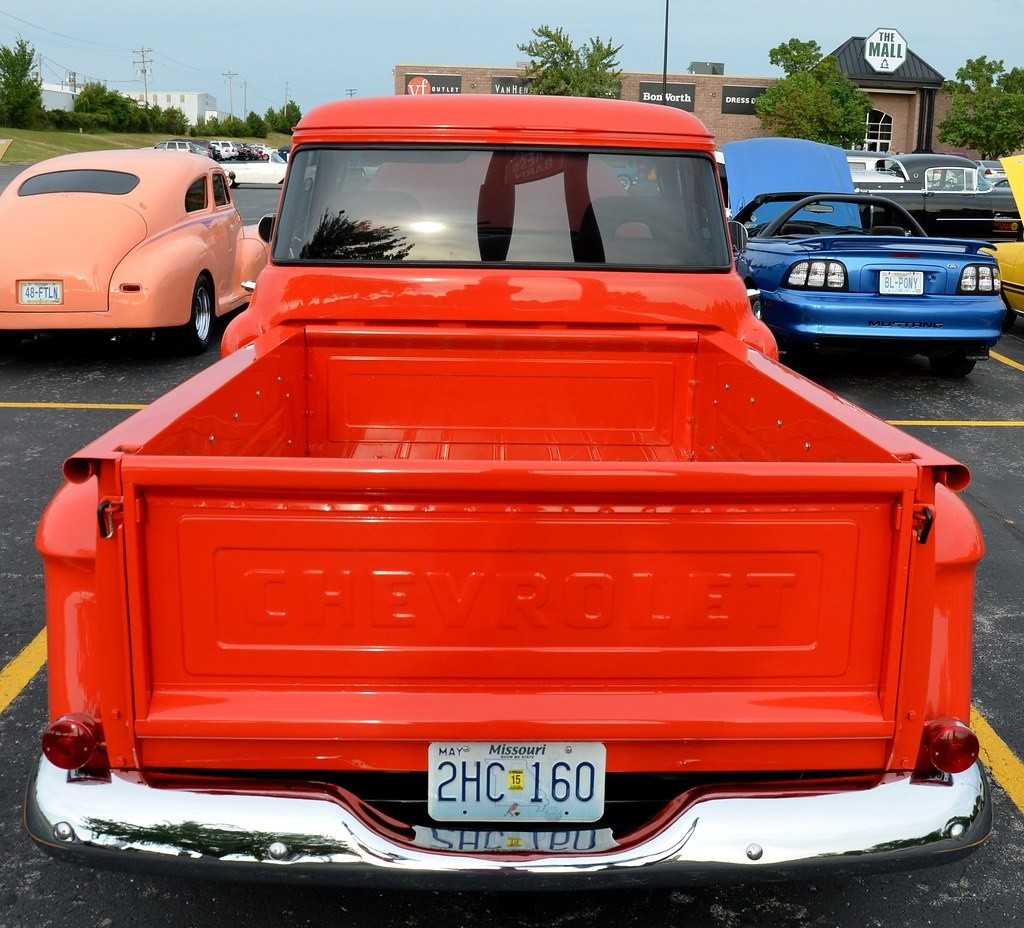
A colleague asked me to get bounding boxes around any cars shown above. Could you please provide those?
[842,148,1024,334]
[0,147,272,357]
[718,135,1009,382]
[139,138,292,161]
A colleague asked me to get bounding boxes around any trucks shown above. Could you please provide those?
[23,92,996,893]
